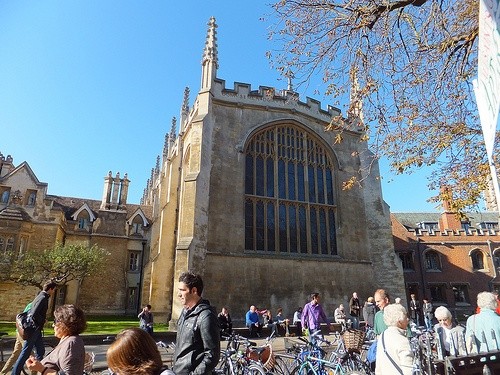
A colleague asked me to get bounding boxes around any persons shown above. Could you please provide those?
[264,311,280,337]
[335,304,352,331]
[0,281,57,375]
[301,293,335,342]
[434,306,466,375]
[138,305,155,337]
[363,297,376,328]
[293,308,302,335]
[106,327,176,375]
[374,289,412,337]
[465,291,500,361]
[218,307,232,340]
[246,306,264,338]
[410,294,421,326]
[349,292,362,329]
[25,304,86,375]
[423,298,434,329]
[375,304,413,375]
[173,272,221,375]
[277,309,295,336]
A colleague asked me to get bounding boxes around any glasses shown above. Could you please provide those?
[438,317,449,321]
[53,319,63,325]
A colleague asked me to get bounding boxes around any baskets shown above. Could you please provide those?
[248,343,276,372]
[284,338,307,349]
[84,352,96,375]
[343,328,366,353]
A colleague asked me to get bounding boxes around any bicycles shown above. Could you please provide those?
[103,322,373,375]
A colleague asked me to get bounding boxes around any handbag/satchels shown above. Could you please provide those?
[15,311,35,340]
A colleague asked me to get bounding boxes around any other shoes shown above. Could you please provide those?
[257,333,260,338]
[277,333,281,337]
